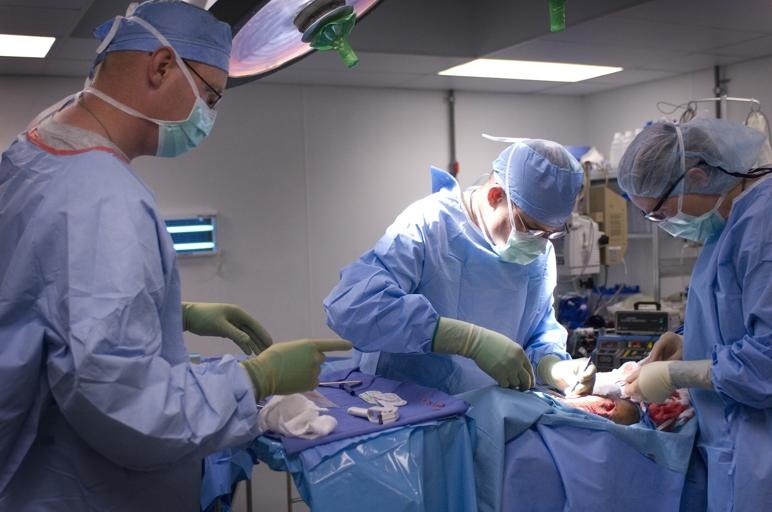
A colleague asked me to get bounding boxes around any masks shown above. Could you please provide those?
[154,64,220,160]
[486,198,548,268]
[657,187,727,246]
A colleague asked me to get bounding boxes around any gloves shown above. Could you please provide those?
[238,337,365,401]
[180,297,272,353]
[432,312,533,391]
[535,357,596,398]
[623,359,709,406]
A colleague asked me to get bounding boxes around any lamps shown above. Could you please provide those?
[201,0,381,90]
[160,212,217,257]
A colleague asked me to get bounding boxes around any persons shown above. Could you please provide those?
[620,111,772,511]
[325,140,595,401]
[560,391,640,426]
[1,0,351,512]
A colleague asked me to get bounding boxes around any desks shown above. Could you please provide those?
[246,365,477,512]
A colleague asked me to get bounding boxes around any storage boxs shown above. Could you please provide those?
[590,185,627,266]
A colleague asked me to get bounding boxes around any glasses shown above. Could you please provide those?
[629,173,682,221]
[514,209,569,240]
[183,54,223,107]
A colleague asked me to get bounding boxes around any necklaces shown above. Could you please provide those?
[467,187,479,230]
[739,178,748,196]
[78,96,113,142]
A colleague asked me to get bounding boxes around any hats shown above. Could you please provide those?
[90,0,234,76]
[490,140,584,225]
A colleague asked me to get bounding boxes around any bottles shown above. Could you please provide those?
[608,129,643,169]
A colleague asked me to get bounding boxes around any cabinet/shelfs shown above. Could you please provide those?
[579,169,703,308]
